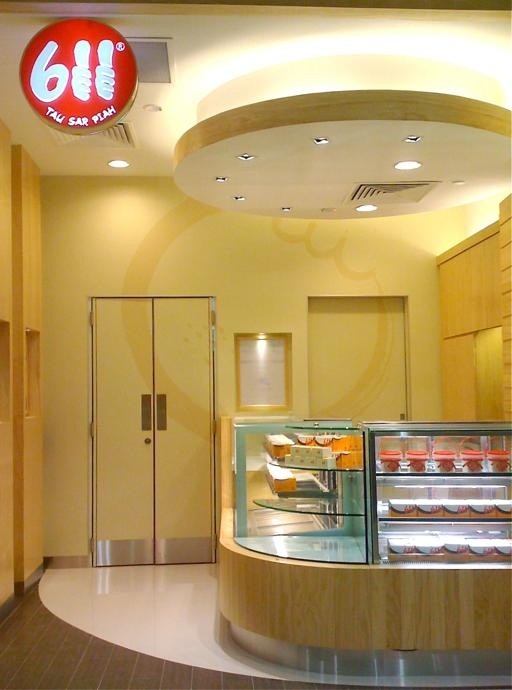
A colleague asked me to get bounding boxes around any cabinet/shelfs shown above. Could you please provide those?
[233,421,373,564]
[358,423,512,565]
